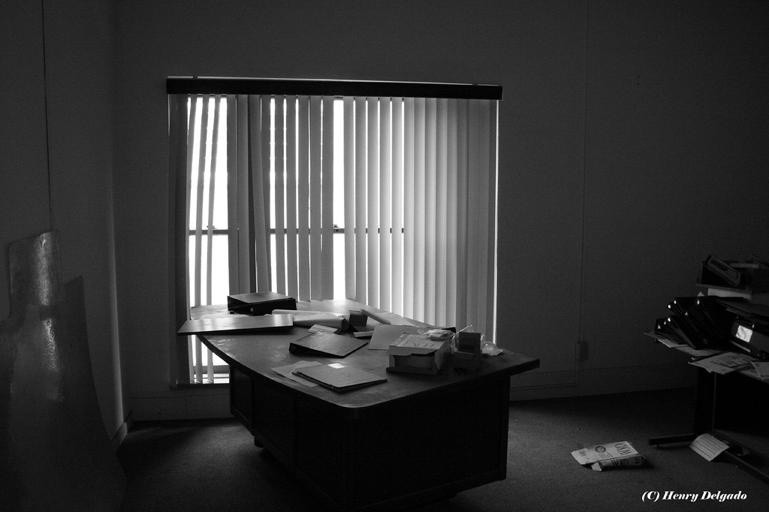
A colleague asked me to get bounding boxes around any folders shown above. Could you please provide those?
[289,330,369,358]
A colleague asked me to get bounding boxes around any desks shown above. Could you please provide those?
[695,356,769,487]
[190,302,537,511]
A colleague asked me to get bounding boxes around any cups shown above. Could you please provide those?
[452,332,481,361]
